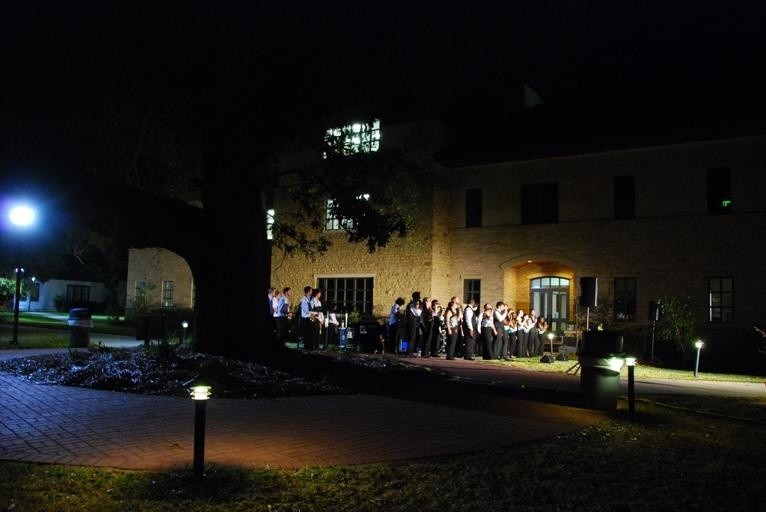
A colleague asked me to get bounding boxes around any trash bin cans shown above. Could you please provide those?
[578,330,626,411]
[67,308,93,348]
[357,315,383,354]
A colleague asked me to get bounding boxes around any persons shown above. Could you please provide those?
[267,286,550,361]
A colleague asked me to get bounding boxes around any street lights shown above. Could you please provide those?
[9,206,35,342]
